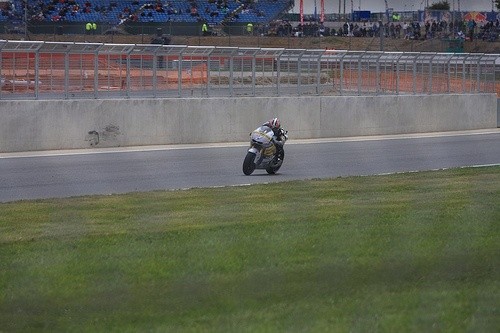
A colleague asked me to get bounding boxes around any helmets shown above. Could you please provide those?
[269,117,280,128]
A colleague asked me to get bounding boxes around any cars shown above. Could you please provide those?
[242,125,285,175]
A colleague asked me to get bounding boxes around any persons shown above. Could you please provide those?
[0,1,499,52]
[260,116,287,163]
